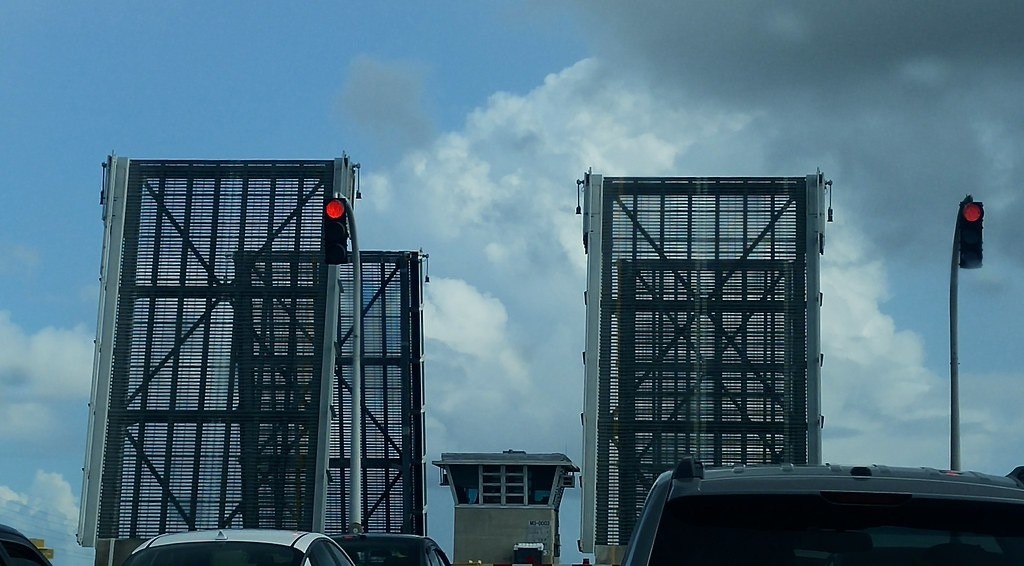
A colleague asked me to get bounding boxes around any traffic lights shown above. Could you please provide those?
[960,201,983,269]
[320,198,348,265]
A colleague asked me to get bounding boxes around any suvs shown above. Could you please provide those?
[620,455,1024,566]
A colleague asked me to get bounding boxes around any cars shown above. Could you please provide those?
[120,526,356,566]
[0,524,53,566]
[326,532,451,566]
